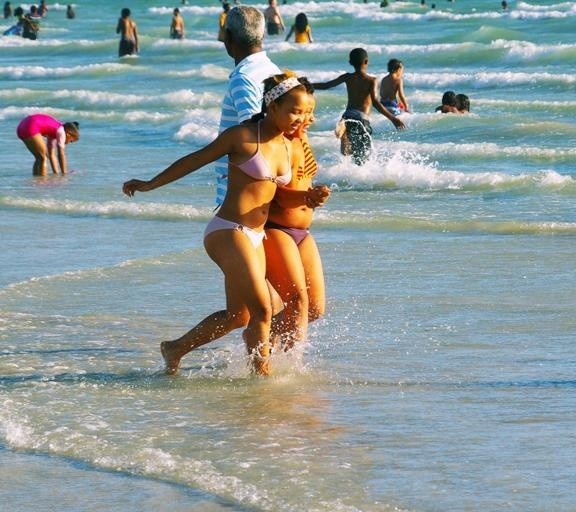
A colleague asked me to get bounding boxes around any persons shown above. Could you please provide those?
[217,3,236,43]
[121,75,307,383]
[283,12,314,46]
[263,0,286,36]
[1,0,51,40]
[170,7,184,39]
[115,7,139,56]
[214,5,282,205]
[362,0,456,11]
[501,0,507,11]
[434,90,457,114]
[306,47,405,166]
[242,77,334,364]
[15,112,82,179]
[375,57,410,117]
[451,93,473,117]
[66,5,76,20]
[218,0,242,5]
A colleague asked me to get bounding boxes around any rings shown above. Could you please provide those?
[319,191,323,197]
